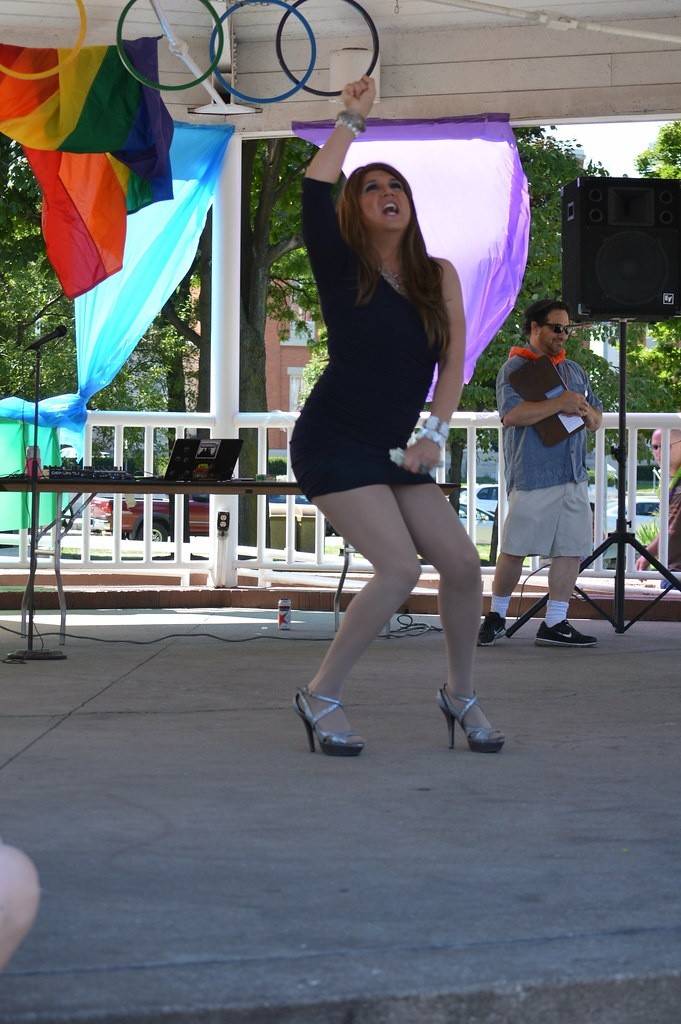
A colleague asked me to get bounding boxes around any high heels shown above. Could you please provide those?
[436,683,504,753]
[291,685,364,757]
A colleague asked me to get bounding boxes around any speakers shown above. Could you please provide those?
[560,176,681,323]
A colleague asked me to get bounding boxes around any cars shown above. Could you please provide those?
[89,491,339,561]
[583,485,661,547]
[443,483,498,548]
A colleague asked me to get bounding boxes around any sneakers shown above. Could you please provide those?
[477,612,506,646]
[535,619,599,648]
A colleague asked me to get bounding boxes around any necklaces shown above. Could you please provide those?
[369,267,414,298]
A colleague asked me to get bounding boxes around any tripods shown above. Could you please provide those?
[504,320,681,639]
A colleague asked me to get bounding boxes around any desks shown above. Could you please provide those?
[0,476,463,660]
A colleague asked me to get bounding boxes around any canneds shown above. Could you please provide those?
[25,446,40,476]
[278,599,291,629]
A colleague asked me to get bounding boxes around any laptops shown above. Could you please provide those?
[134,439,244,480]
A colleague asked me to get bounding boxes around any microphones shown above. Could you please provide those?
[23,325,68,352]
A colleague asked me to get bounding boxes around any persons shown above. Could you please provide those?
[476,298,604,647]
[634,429,681,591]
[287,72,508,757]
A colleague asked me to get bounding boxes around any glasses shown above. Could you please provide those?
[533,318,571,334]
[652,441,681,451]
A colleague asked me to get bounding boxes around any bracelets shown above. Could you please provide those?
[334,108,366,138]
[416,415,450,448]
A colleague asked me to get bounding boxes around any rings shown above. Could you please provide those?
[418,464,430,474]
